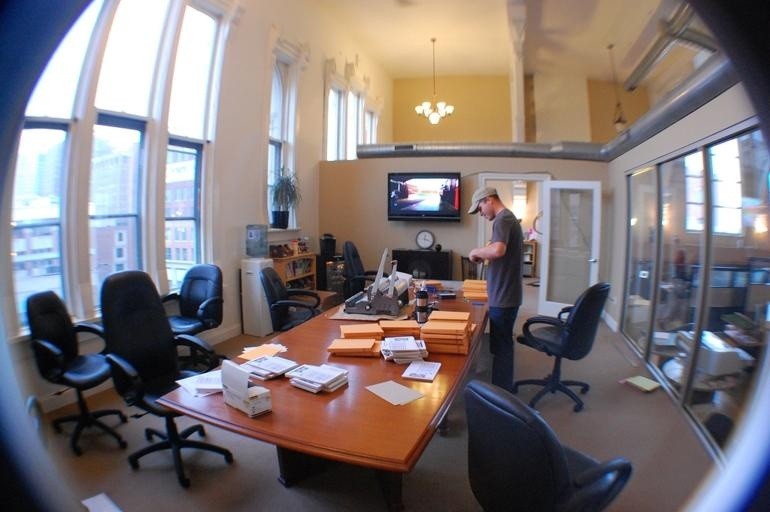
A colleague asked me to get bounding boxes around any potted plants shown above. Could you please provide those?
[270,171,302,229]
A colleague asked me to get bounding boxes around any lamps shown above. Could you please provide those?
[613,103,628,131]
[414,101,454,126]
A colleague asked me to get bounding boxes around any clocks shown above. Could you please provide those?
[414,230,435,249]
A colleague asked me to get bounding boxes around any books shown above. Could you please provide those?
[423,280,448,291]
[328,339,380,358]
[625,376,664,394]
[464,280,491,299]
[340,324,384,336]
[421,311,476,355]
[381,336,428,365]
[239,355,298,381]
[380,320,421,337]
[402,360,441,381]
[285,364,348,394]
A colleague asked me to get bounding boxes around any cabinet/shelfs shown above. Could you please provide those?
[272,250,317,291]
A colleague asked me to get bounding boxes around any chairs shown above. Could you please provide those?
[158,263,223,359]
[464,379,633,512]
[513,282,610,414]
[343,241,388,299]
[99,270,234,489]
[25,290,128,459]
[259,267,321,332]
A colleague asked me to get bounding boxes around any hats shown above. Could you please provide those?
[468,185,498,215]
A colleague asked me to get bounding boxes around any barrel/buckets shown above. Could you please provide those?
[245,224,268,257]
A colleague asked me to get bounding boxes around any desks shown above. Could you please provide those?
[154,279,489,502]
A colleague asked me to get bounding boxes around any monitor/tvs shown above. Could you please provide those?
[388,171,462,223]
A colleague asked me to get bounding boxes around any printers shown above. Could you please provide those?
[343,246,411,319]
[675,329,745,377]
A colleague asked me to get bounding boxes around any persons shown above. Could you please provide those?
[470,186,524,389]
